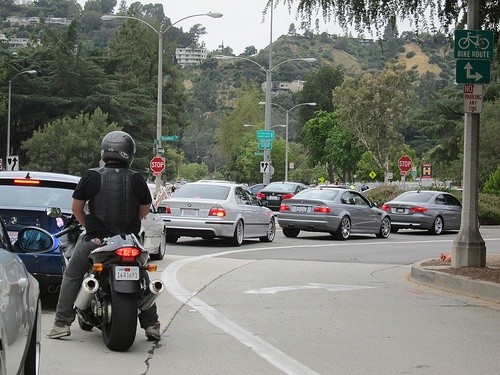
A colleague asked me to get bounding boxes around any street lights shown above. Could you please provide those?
[100,11,224,201]
[7,70,37,158]
[214,54,318,187]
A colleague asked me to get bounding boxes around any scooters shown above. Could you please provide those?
[46,207,164,352]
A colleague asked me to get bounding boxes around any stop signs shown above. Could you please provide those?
[149,157,166,174]
[398,154,413,173]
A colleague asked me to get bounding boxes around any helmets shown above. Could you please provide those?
[101,130,135,168]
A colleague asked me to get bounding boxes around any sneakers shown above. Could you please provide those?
[145,325,161,339]
[45,323,70,337]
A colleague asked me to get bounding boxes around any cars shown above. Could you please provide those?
[139,180,276,259]
[381,188,480,235]
[247,181,392,240]
[0,170,90,307]
[0,215,42,375]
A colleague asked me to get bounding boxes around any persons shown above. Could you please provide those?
[45,131,160,341]
